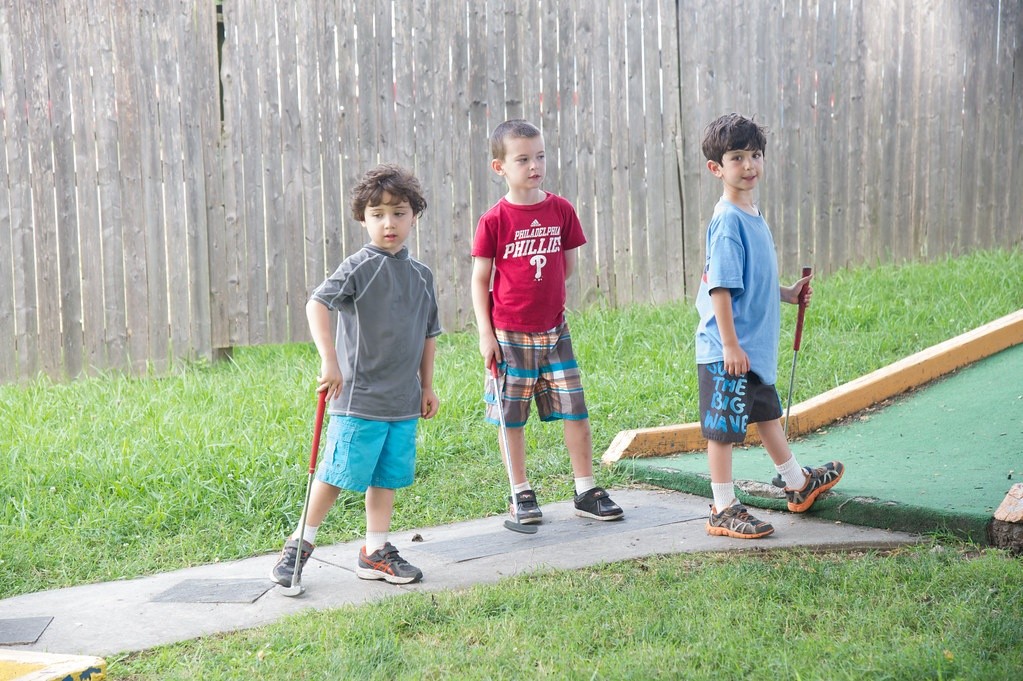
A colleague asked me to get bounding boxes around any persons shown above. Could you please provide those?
[471,119,624,525]
[694,110,845,538]
[269,163,443,584]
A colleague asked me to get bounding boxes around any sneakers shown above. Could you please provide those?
[784,460,845,512]
[573,487,624,520]
[707,497,774,539]
[356,542,423,585]
[508,490,543,524]
[270,535,314,588]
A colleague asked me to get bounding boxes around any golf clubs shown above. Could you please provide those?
[773,266,813,490]
[492,352,539,535]
[284,374,330,597]
